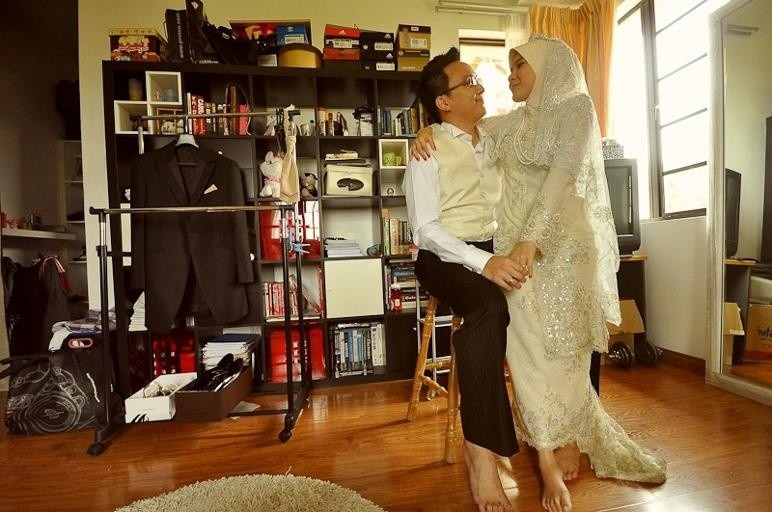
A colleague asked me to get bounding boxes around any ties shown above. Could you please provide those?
[280,105,300,206]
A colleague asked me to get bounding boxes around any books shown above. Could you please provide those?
[187,87,237,135]
[202,333,260,370]
[319,107,347,137]
[382,209,419,256]
[128,292,147,331]
[265,275,299,319]
[325,239,363,258]
[329,322,386,379]
[385,259,430,313]
[378,98,425,137]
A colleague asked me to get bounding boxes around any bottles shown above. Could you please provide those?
[391,276,401,313]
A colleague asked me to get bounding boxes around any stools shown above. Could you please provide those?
[409,293,465,466]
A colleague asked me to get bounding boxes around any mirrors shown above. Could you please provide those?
[705,0,771,408]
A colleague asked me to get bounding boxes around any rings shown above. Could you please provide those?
[508,277,515,284]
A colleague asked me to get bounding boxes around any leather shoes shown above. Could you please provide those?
[191,353,244,391]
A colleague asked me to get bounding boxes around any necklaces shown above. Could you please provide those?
[512,115,555,167]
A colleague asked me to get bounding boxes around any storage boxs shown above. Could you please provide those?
[600,297,646,368]
[326,163,373,197]
[722,301,772,367]
[124,367,253,424]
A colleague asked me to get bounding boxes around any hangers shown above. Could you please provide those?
[174,114,200,149]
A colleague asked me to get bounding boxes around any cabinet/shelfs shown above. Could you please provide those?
[105,56,453,395]
[59,139,87,306]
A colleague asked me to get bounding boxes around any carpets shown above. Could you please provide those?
[112,471,391,512]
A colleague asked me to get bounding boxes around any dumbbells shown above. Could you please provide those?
[608,338,663,368]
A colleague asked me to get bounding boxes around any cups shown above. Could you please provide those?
[382,151,396,166]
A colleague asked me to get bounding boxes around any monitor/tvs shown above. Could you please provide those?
[604,158,641,257]
[726,168,741,259]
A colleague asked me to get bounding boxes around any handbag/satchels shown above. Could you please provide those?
[165,0,221,65]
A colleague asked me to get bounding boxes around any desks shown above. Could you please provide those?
[619,254,649,368]
[725,257,757,365]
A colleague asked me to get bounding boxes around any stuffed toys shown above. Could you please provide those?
[259,151,284,199]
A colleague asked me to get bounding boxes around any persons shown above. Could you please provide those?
[399,42,602,510]
[406,35,665,511]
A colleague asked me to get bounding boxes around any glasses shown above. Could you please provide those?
[440,73,483,96]
